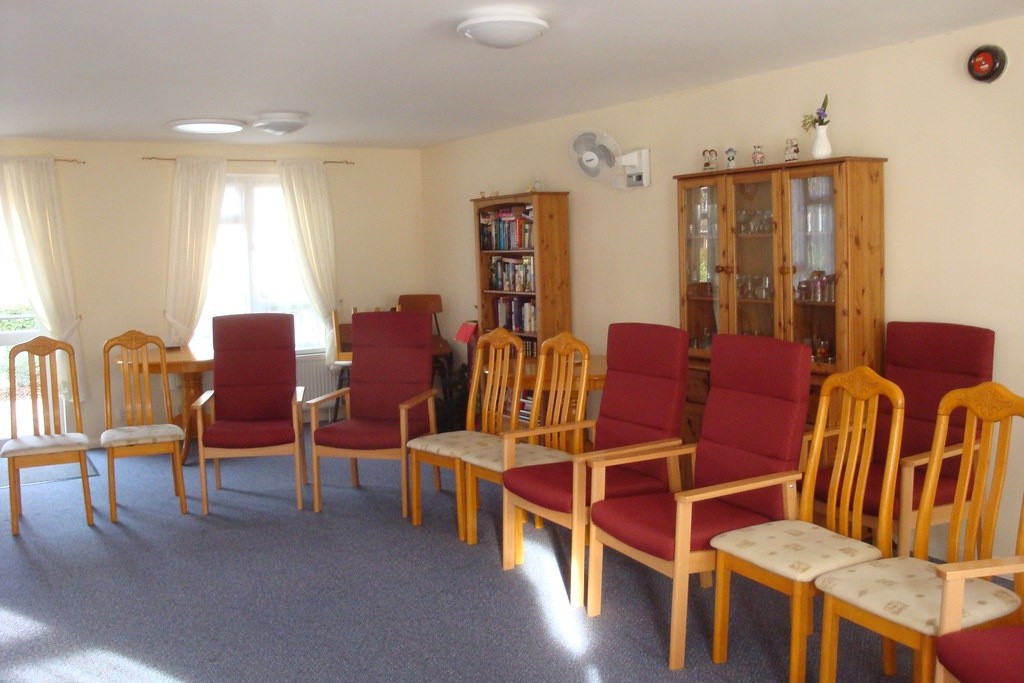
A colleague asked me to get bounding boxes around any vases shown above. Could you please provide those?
[812,125,832,160]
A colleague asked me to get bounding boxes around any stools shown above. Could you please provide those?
[330,304,382,361]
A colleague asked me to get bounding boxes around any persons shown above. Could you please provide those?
[785,140,800,161]
[702,150,719,167]
[726,149,739,169]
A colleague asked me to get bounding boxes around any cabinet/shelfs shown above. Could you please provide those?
[672,155,889,474]
[469,191,571,447]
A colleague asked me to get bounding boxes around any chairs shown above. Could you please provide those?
[585,332,813,671]
[396,293,452,385]
[325,324,354,421]
[189,313,310,515]
[932,554,1024,683]
[460,332,589,565]
[405,329,530,546]
[100,329,189,522]
[500,321,714,606]
[815,321,995,585]
[307,311,442,519]
[710,366,906,683]
[0,335,95,536]
[815,382,1024,683]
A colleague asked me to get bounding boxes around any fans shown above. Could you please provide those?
[564,130,652,192]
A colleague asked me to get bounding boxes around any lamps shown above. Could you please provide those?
[251,111,309,136]
[457,9,550,49]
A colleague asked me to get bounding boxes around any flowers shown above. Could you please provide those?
[803,93,831,132]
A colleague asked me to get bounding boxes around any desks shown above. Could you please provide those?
[482,355,613,454]
[114,347,214,467]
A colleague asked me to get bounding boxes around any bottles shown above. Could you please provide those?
[804,337,834,363]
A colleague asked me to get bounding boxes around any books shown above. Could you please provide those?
[480,211,535,251]
[501,390,513,418]
[510,341,537,359]
[491,298,536,331]
[519,394,543,426]
[488,256,535,293]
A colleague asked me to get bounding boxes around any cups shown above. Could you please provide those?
[736,273,773,300]
[688,271,712,297]
[689,327,712,350]
[798,268,835,302]
[736,207,772,234]
[738,328,774,336]
